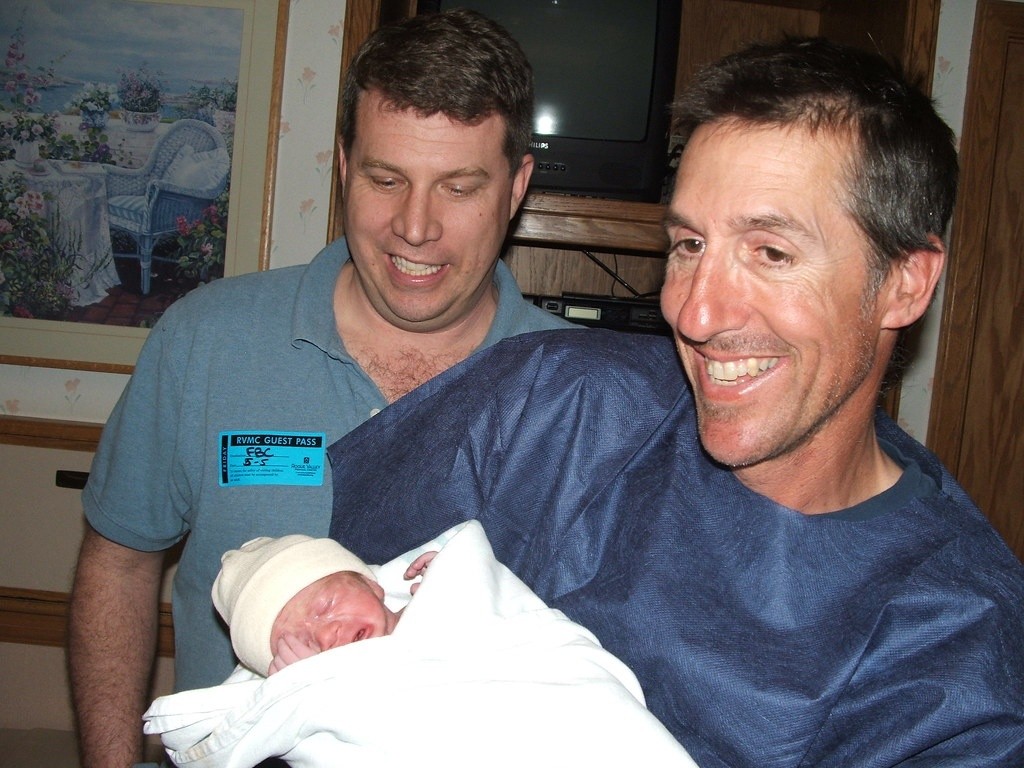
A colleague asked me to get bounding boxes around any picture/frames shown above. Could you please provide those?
[0,1,299,463]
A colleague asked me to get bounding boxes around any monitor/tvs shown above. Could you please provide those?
[417,0,683,203]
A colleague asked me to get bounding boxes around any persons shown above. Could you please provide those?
[142,520,702,768]
[325,27,1024,768]
[66,6,596,768]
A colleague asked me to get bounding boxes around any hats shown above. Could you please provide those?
[212,535,379,682]
